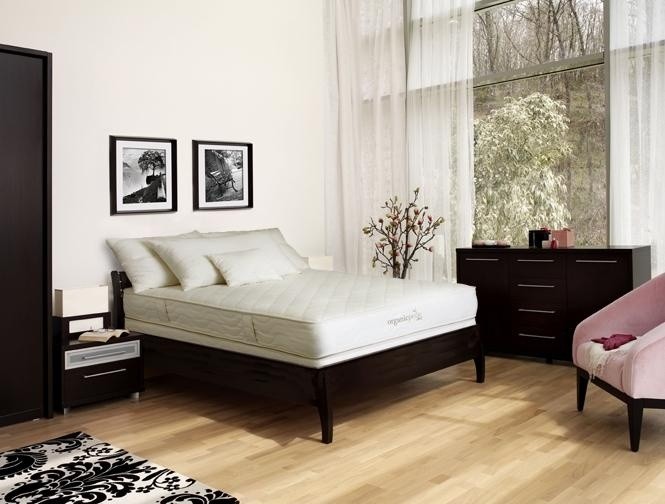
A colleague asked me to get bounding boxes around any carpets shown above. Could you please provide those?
[0,431,239,504]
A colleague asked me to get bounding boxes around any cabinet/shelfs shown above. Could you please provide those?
[455,244,653,369]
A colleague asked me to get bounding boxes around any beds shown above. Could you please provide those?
[109,269,485,444]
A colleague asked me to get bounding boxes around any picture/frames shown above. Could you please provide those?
[108,135,178,216]
[191,139,253,212]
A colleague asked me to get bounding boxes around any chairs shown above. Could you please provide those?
[571,270,664,452]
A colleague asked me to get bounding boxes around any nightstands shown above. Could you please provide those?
[52,284,143,414]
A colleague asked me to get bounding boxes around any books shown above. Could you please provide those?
[78,327,130,344]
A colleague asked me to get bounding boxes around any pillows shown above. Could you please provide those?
[138,228,309,292]
[103,231,202,292]
[206,247,284,289]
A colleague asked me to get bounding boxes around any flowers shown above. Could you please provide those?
[362,188,445,279]
